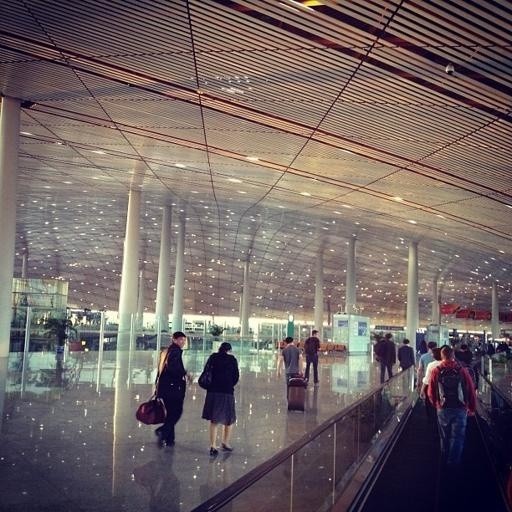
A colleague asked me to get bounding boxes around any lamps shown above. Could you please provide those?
[446,63,454,75]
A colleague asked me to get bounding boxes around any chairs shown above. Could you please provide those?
[276,341,346,353]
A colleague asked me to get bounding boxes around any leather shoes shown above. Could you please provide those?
[210,448,218,455]
[222,443,232,451]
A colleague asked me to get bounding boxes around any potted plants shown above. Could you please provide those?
[42,317,78,353]
[210,324,223,341]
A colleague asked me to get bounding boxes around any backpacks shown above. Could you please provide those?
[438,367,466,408]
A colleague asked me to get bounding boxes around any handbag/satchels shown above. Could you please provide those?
[198,369,214,389]
[136,400,165,424]
[156,377,186,399]
[289,378,305,385]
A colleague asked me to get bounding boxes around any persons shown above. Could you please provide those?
[148,448,181,511]
[201,342,239,457]
[304,331,320,387]
[275,335,304,402]
[199,457,233,512]
[154,331,192,449]
[372,331,510,482]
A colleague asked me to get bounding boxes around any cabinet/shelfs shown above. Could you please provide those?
[10,278,69,339]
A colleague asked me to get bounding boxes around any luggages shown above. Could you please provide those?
[288,386,307,411]
